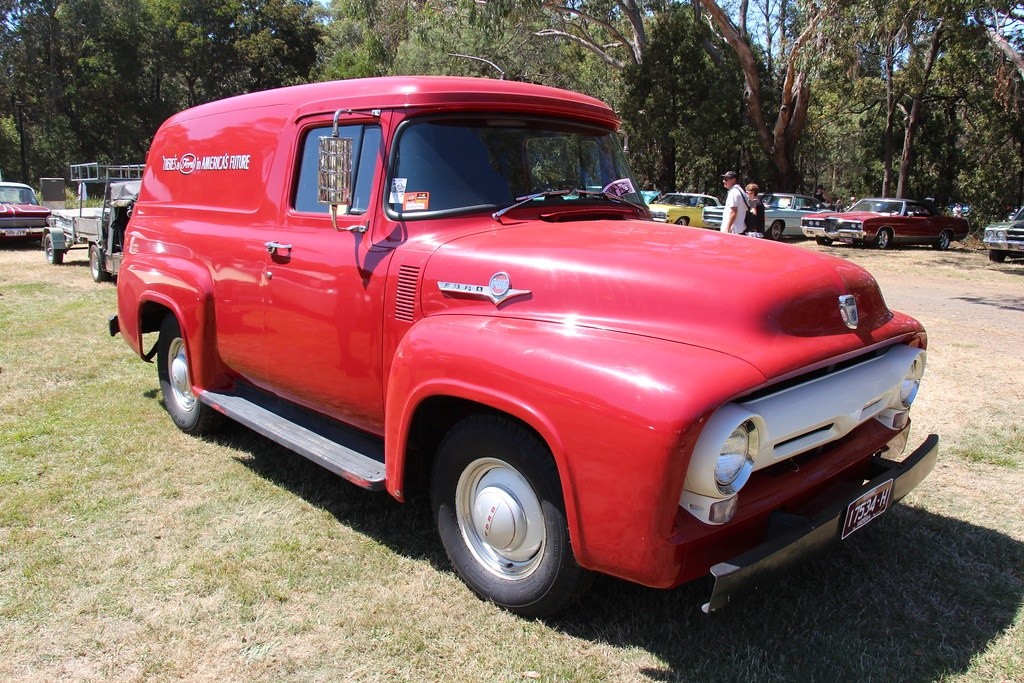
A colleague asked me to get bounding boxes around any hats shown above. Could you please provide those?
[720,171,737,177]
[817,185,824,189]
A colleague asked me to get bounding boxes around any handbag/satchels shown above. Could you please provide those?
[744,211,758,231]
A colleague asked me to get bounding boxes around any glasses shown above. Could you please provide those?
[722,178,733,180]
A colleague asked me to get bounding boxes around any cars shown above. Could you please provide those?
[800,199,969,251]
[561,185,602,200]
[701,191,839,241]
[109,73,940,622]
[983,206,1024,263]
[646,192,724,228]
[0,182,52,242]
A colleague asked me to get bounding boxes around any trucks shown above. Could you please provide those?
[42,162,142,284]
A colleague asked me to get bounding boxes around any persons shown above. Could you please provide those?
[953,203,963,219]
[75,177,88,201]
[813,184,855,213]
[719,171,750,235]
[743,183,765,239]
